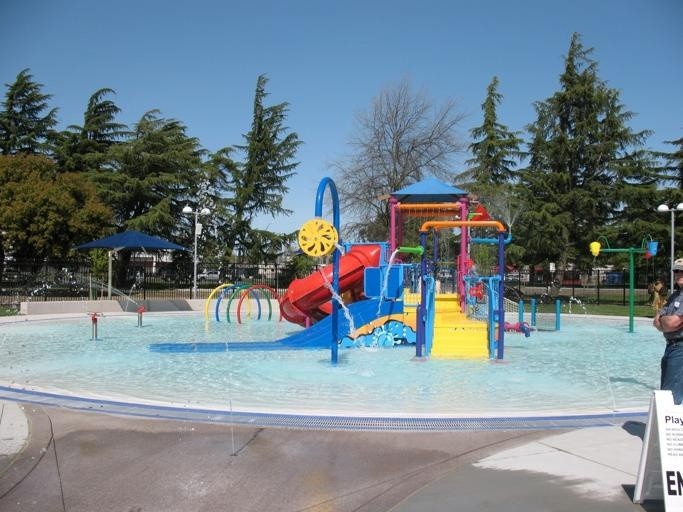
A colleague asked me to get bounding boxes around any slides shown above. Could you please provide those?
[146,301,402,354]
[280,245,381,327]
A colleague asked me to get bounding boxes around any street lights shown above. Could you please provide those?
[180,205,210,299]
[657,201,682,294]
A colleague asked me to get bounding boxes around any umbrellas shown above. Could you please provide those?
[72,230,193,300]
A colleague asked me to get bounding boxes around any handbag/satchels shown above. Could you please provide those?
[659,286,667,296]
[648,286,654,294]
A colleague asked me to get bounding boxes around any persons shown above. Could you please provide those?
[651,277,666,310]
[651,255,682,407]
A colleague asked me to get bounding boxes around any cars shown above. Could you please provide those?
[1,266,89,297]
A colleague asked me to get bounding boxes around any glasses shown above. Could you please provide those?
[673,270,683,273]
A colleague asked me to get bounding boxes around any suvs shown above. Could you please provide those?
[161,270,187,281]
[197,270,220,283]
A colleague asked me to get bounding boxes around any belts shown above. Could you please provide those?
[666,337,683,345]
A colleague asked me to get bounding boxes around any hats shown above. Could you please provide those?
[673,258,683,270]
